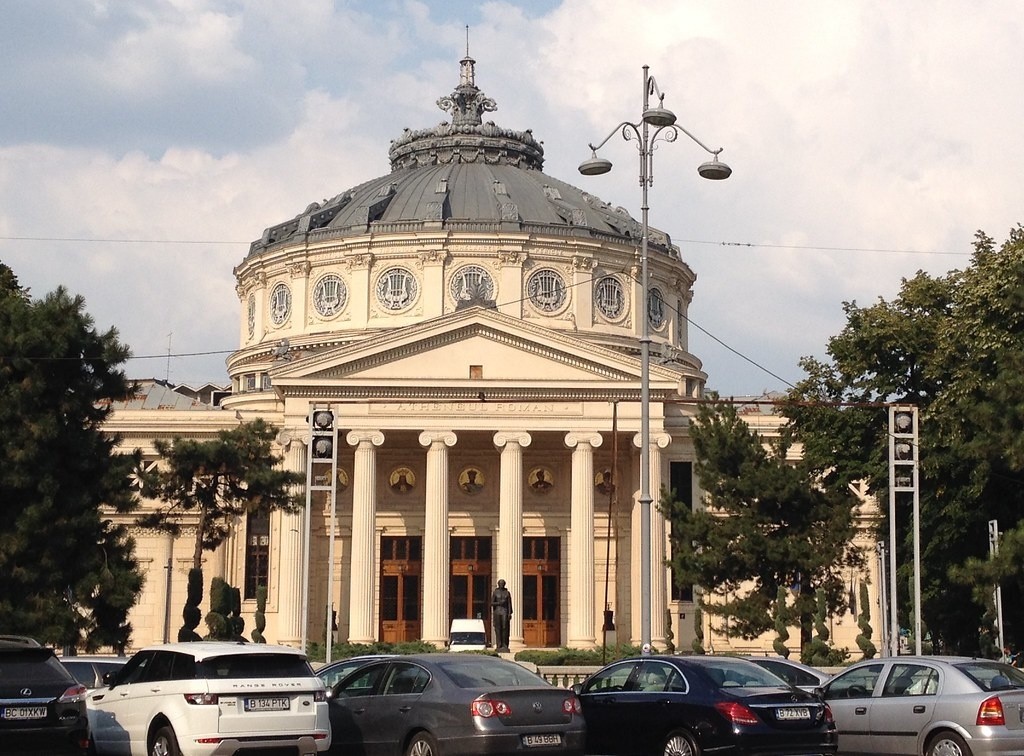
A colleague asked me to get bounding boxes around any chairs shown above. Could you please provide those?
[393,670,423,693]
[893,676,913,695]
[643,666,672,692]
[991,675,1009,688]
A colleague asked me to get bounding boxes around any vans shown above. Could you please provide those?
[444,619,493,653]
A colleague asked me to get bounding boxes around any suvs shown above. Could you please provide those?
[0,633,92,756]
[85,640,335,756]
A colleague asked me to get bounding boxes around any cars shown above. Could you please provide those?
[566,654,840,756]
[812,655,1024,756]
[743,656,865,700]
[57,654,147,697]
[314,653,401,693]
[311,652,589,756]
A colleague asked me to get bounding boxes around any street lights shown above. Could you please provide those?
[577,63,733,662]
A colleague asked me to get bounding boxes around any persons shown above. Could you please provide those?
[489,579,512,648]
[1003,646,1017,668]
